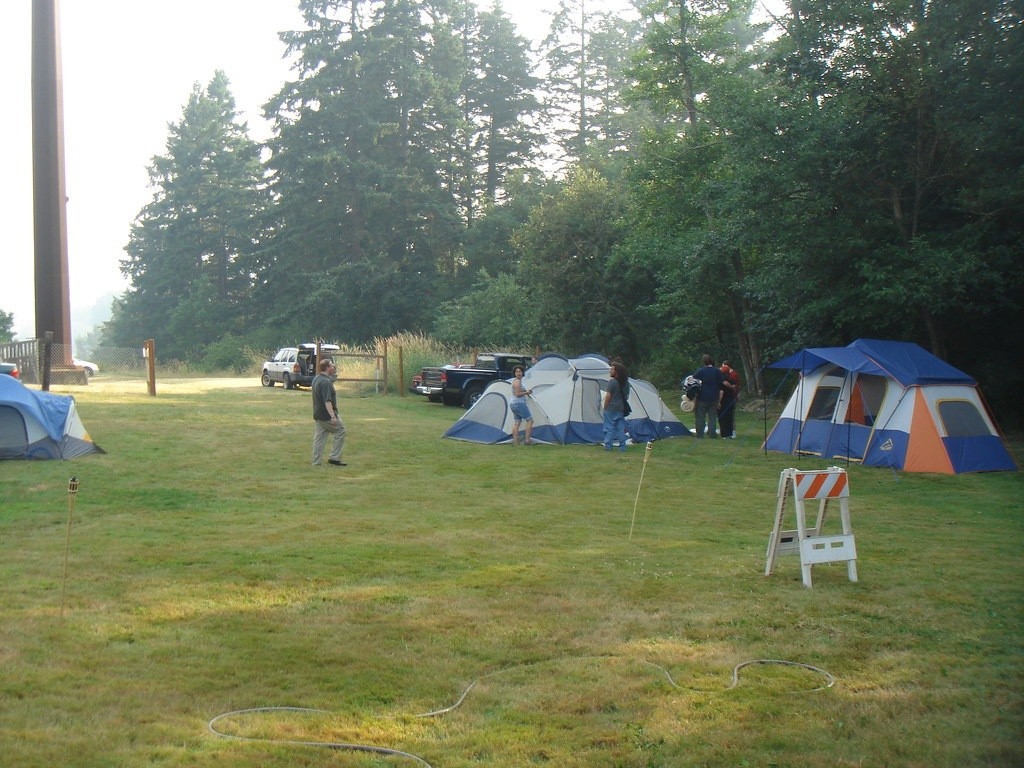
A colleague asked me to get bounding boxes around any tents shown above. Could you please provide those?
[0,374,108,462]
[762,338,1017,474]
[445,353,693,446]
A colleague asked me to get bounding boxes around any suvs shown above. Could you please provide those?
[261,343,340,390]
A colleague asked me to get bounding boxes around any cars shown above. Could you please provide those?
[72,358,99,377]
[409,363,476,403]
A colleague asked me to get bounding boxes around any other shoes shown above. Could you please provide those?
[328,459,347,466]
[512,443,520,447]
[525,442,537,445]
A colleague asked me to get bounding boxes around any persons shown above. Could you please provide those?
[510,365,537,445]
[688,354,740,440]
[312,359,347,465]
[602,361,632,451]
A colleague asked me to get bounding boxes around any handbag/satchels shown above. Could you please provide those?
[623,399,632,416]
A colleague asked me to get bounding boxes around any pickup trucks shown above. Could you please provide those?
[417,353,534,410]
